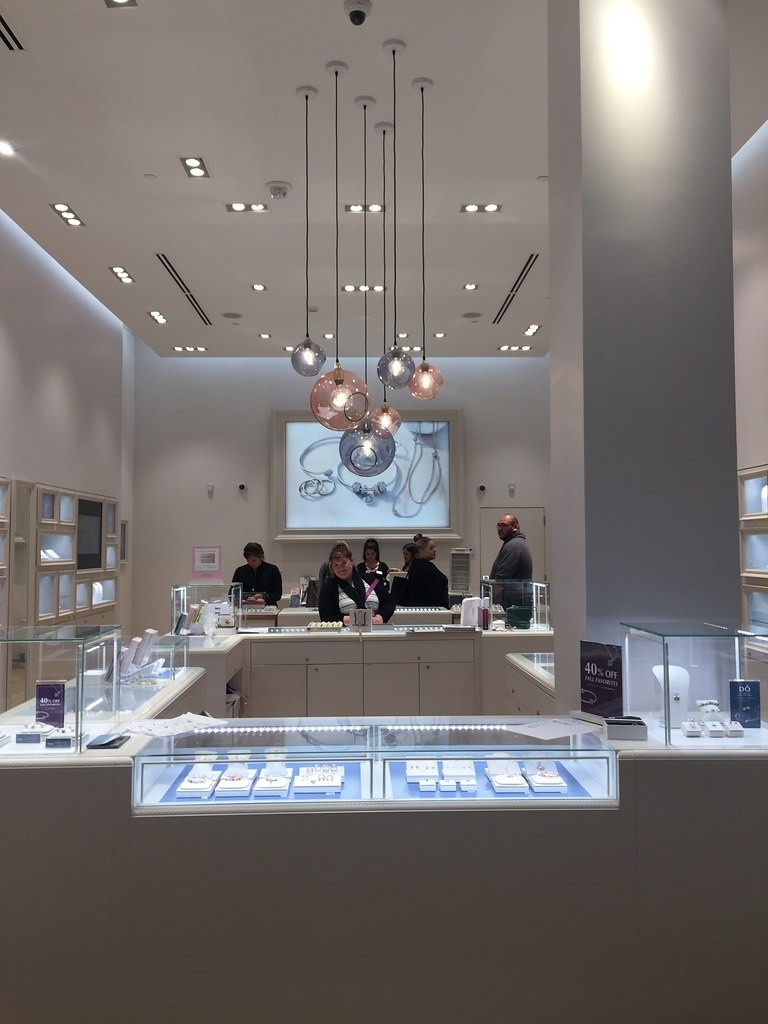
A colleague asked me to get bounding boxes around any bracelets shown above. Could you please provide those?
[36,710,49,720]
[300,437,400,503]
[538,770,558,779]
[580,687,597,705]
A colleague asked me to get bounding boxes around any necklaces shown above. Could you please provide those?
[604,644,618,666]
[51,683,62,697]
[393,421,442,518]
[662,667,681,702]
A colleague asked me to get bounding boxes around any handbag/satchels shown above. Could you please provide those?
[391,572,410,606]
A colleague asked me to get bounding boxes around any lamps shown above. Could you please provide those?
[291,39,443,478]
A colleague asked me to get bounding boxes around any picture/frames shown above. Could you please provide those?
[268,401,462,546]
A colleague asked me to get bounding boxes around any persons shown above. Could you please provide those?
[228,541,283,608]
[487,514,532,616]
[410,533,451,609]
[317,538,395,622]
[389,543,416,574]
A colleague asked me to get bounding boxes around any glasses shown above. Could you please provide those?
[496,524,513,528]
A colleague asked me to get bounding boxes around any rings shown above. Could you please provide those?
[297,478,334,498]
[302,763,338,784]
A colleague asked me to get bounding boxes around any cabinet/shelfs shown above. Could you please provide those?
[0,462,768,1022]
